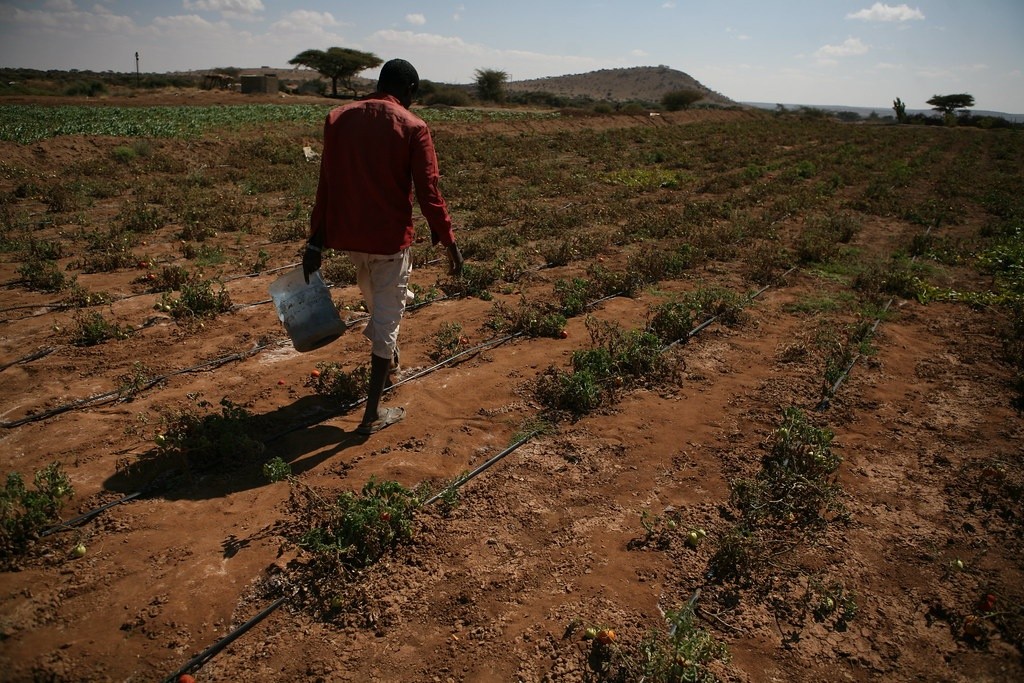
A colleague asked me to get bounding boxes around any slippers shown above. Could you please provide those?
[357,406,406,433]
[388,347,401,374]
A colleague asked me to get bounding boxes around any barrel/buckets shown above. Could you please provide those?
[268,266,347,353]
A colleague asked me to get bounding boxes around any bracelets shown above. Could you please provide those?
[306,243,322,251]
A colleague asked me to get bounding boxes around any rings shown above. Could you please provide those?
[314,266,318,270]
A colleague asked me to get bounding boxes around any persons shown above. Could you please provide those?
[301,59,464,435]
